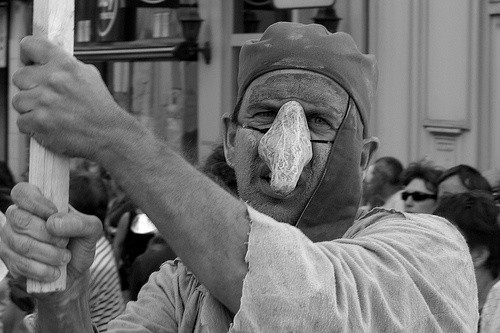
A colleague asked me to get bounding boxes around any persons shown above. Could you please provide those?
[399,163,443,214]
[0,144,239,332]
[359,166,375,210]
[1,21,479,333]
[370,156,407,211]
[434,165,492,208]
[431,190,500,333]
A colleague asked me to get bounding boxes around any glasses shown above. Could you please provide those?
[402,192,437,201]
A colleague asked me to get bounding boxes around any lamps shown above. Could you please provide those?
[177,6,210,64]
[311,5,342,34]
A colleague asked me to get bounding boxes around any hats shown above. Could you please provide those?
[235,21,379,139]
